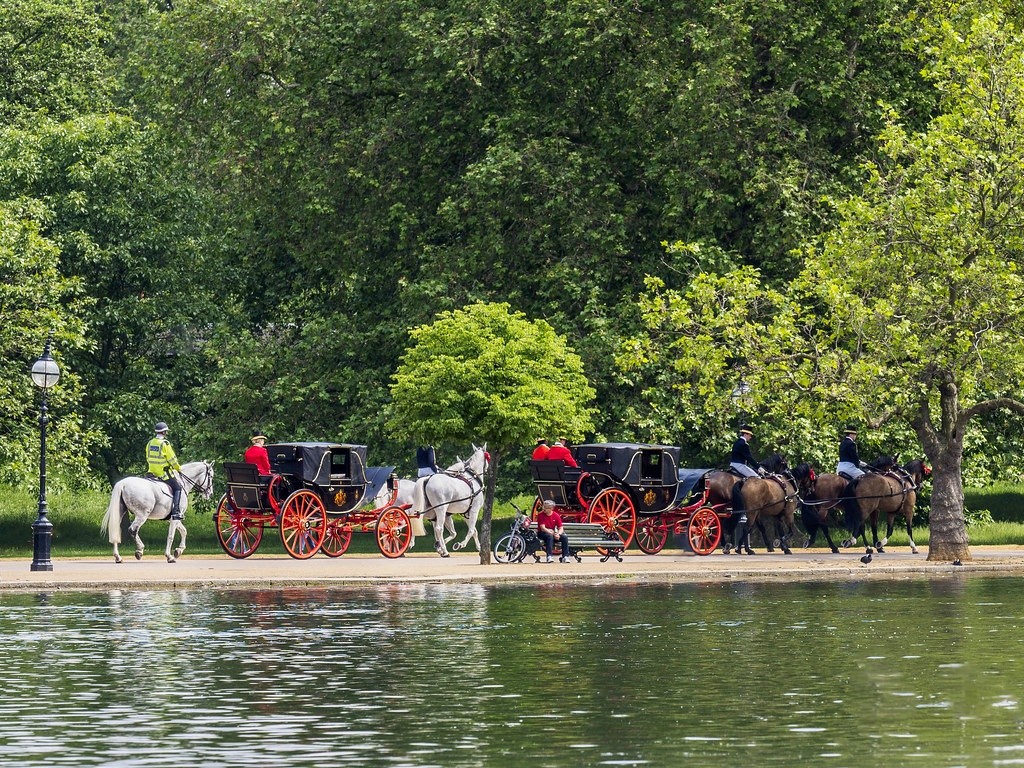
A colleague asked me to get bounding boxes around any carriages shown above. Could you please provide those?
[528,442,818,556]
[212,442,491,559]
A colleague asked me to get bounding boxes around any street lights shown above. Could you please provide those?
[31,345,60,571]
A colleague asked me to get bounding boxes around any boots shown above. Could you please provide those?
[172,489,184,520]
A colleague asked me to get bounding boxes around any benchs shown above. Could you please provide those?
[223,462,280,510]
[527,459,586,507]
[529,522,625,563]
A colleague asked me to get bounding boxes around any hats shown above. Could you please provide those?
[250,429,267,440]
[843,426,857,433]
[739,425,754,436]
[537,435,549,441]
[557,433,569,440]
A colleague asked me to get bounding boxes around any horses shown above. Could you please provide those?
[374,455,465,558]
[412,441,489,559]
[101,458,216,564]
[684,446,816,555]
[841,457,926,564]
[803,452,901,554]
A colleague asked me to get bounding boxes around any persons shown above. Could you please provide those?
[537,500,570,563]
[547,435,578,468]
[533,436,550,460]
[146,422,184,519]
[245,432,270,475]
[417,445,437,476]
[730,426,765,477]
[838,425,867,479]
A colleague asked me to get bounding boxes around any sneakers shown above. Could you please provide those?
[547,555,554,563]
[561,556,571,563]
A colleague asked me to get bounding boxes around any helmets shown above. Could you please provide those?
[155,422,170,432]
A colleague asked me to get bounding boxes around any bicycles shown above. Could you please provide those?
[493,502,532,564]
